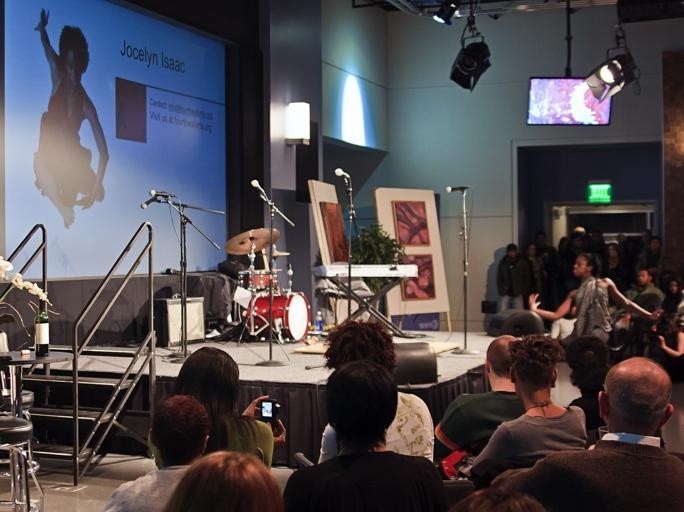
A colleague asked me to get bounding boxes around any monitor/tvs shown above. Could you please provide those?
[525,76,614,126]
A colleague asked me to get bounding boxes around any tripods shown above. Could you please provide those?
[236,242,285,347]
[304,178,391,370]
[236,243,269,347]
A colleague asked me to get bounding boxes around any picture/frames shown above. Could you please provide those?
[391,200,431,248]
[397,253,436,303]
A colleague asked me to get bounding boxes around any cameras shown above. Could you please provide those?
[260,399,277,420]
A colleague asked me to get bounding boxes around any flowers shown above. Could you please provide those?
[0,256,54,353]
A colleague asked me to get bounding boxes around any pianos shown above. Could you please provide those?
[311,265,419,278]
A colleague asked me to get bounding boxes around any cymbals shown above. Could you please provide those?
[261,251,291,257]
[225,228,280,255]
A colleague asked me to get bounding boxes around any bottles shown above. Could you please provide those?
[316,311,322,331]
[35,298,49,359]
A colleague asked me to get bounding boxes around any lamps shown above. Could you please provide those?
[432,0,462,26]
[584,2,642,104]
[286,102,311,147]
[448,0,492,92]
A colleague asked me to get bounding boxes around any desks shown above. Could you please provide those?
[0,350,74,512]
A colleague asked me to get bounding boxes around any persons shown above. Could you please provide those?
[662,279,684,327]
[541,249,553,270]
[658,314,684,357]
[559,236,568,254]
[434,335,533,481]
[641,230,650,244]
[491,357,683,512]
[575,226,586,233]
[103,394,212,512]
[448,487,547,512]
[566,334,665,449]
[148,347,287,472]
[318,319,434,464]
[529,253,663,371]
[568,233,590,290]
[163,450,283,512]
[458,334,587,478]
[31,7,110,229]
[635,236,665,287]
[601,242,627,283]
[405,265,434,299]
[283,359,449,512]
[628,268,666,322]
[524,242,548,308]
[497,243,529,313]
[535,230,557,256]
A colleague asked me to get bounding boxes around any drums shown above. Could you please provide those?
[239,271,271,290]
[247,291,311,342]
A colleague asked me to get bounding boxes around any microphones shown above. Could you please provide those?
[149,189,175,197]
[334,167,351,178]
[139,196,155,209]
[251,180,269,201]
[446,186,472,193]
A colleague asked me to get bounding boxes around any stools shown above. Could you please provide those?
[0,389,45,512]
[0,415,39,512]
